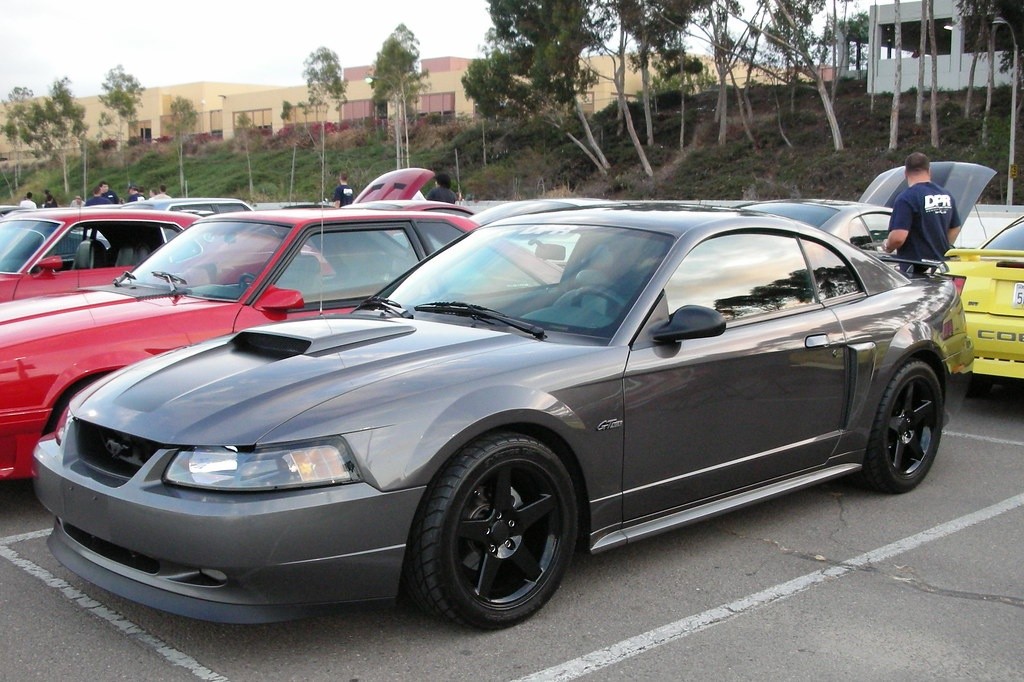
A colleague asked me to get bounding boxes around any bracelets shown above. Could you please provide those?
[883,241,892,252]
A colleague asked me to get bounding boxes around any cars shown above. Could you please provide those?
[934,215,1024,381]
[30,204,975,623]
[1,167,436,297]
[1,208,565,471]
[476,162,999,268]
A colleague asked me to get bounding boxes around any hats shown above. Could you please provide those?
[128,185,138,192]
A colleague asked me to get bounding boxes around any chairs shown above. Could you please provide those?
[286,253,323,295]
[113,242,151,268]
[573,245,620,315]
[71,239,108,270]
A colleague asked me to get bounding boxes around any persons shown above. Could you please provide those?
[19,189,57,208]
[881,152,961,273]
[426,173,456,204]
[333,172,354,208]
[70,180,172,207]
